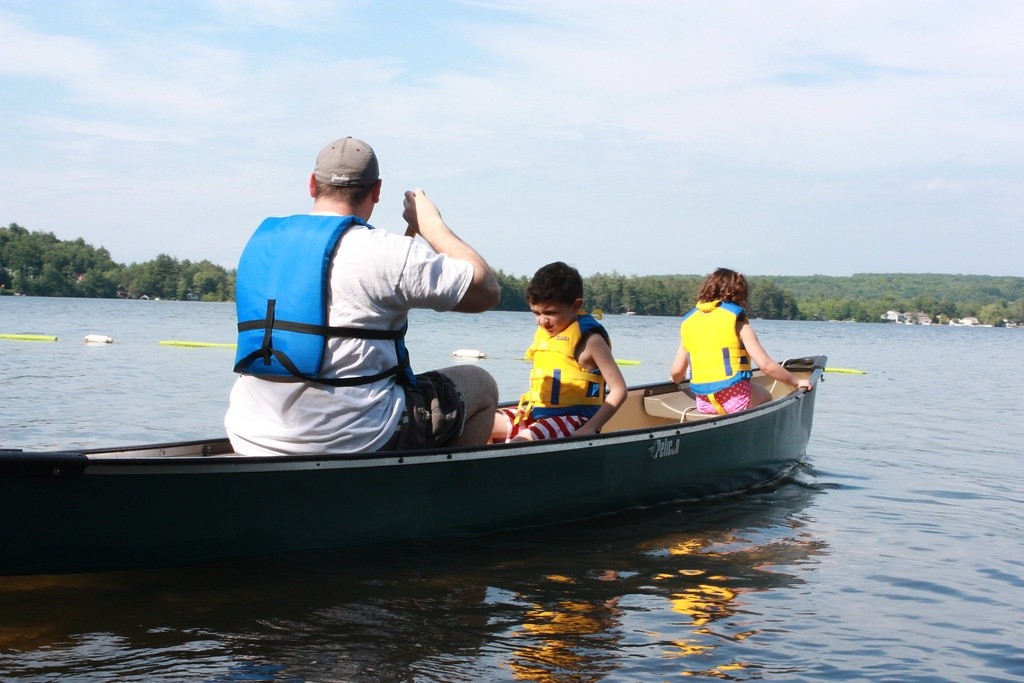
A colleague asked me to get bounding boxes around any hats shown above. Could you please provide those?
[313,136,379,186]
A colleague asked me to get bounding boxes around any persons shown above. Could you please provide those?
[224,137,499,456]
[487,261,628,445]
[671,268,812,414]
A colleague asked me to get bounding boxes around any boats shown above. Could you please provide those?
[1,356,829,584]
